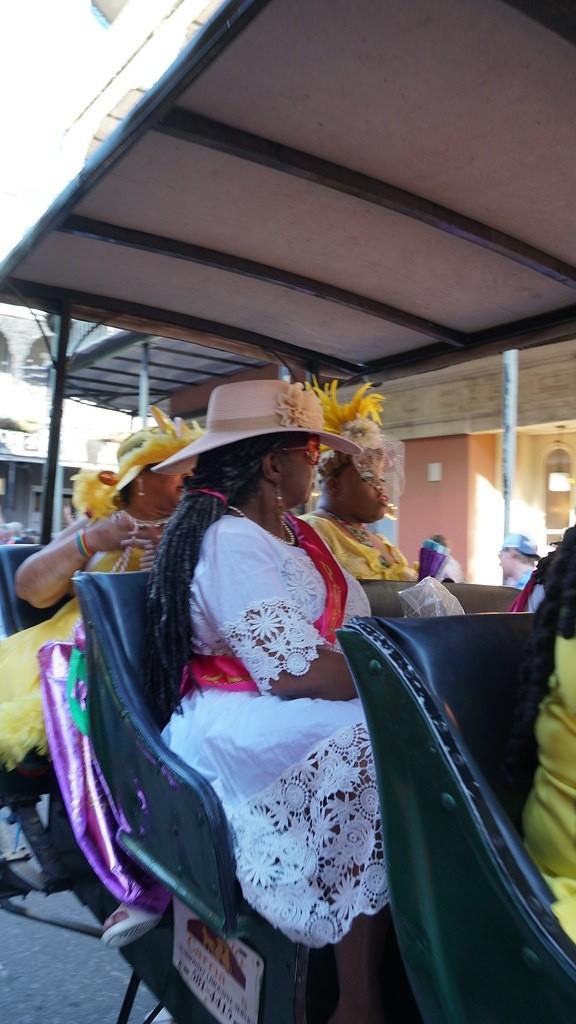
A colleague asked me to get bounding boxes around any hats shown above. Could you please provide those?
[150,381,364,474]
[501,535,541,560]
[113,427,168,491]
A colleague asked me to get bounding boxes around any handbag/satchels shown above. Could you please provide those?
[37,624,170,914]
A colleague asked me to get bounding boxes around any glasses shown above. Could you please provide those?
[276,435,321,464]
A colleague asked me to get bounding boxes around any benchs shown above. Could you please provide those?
[74,568,528,939]
[335,611,576,1021]
[0,541,98,800]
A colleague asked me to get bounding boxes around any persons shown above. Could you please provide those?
[295,420,419,582]
[513,523,575,943]
[1,507,75,548]
[419,536,459,582]
[148,376,400,1024]
[496,538,548,613]
[15,425,205,950]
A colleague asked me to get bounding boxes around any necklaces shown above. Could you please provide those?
[227,502,297,549]
[316,506,394,569]
[115,510,176,529]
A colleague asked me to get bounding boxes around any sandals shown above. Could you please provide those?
[101,902,163,949]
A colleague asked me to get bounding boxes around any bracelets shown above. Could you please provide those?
[74,525,98,559]
